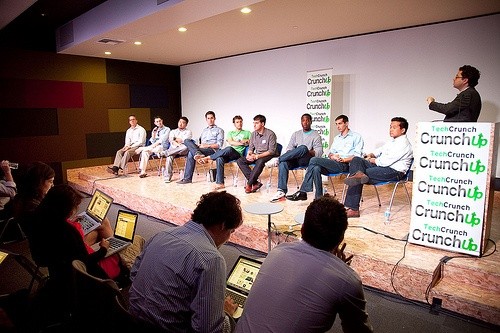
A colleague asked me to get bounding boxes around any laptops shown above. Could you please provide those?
[72,188,114,236]
[225,255,263,319]
[89,210,139,259]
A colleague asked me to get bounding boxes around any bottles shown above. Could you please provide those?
[324,186,328,194]
[0,162,19,169]
[266,181,270,189]
[162,166,165,177]
[384,208,390,224]
[179,170,184,180]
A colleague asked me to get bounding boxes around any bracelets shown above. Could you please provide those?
[256,156,258,159]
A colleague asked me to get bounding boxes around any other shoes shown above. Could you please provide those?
[163,176,171,182]
[194,154,205,160]
[197,157,209,165]
[139,172,147,178]
[108,166,126,176]
[176,178,192,184]
[212,183,225,191]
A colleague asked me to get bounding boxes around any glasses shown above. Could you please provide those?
[455,75,464,79]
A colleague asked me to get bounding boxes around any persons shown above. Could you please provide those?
[0,159,132,290]
[234,193,373,333]
[177,111,224,184]
[428,65,482,122]
[106,115,147,175]
[236,114,277,193]
[288,115,363,201]
[344,117,413,218]
[129,190,243,333]
[265,114,322,202]
[197,116,251,190]
[138,117,171,178]
[157,117,192,183]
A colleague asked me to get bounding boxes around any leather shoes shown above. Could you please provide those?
[244,181,263,193]
[286,191,308,201]
[344,172,369,186]
[345,208,360,218]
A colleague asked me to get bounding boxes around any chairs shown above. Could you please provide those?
[122,139,415,221]
[0,200,161,333]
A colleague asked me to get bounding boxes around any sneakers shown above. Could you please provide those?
[265,158,279,169]
[270,190,286,203]
